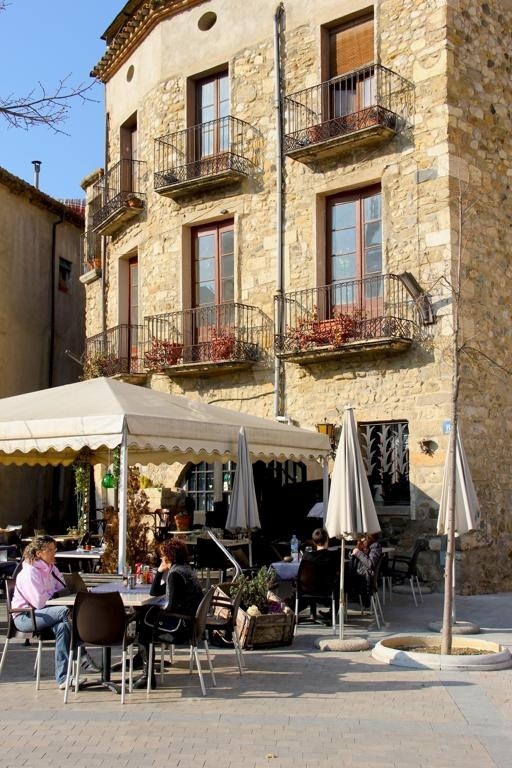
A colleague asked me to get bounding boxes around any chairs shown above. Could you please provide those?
[63,589,135,704]
[346,554,389,631]
[145,585,219,696]
[381,537,426,608]
[0,520,254,647]
[294,558,338,636]
[189,576,248,676]
[1,579,57,692]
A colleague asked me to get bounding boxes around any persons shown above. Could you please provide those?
[112,539,205,689]
[280,527,339,620]
[10,534,104,691]
[317,534,382,622]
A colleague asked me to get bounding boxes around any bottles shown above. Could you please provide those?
[135,562,157,584]
[290,535,299,562]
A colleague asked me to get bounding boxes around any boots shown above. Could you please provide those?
[126,646,144,669]
[132,656,157,689]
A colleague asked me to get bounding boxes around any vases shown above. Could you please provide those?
[154,343,184,365]
[302,318,358,341]
[305,124,326,143]
[210,339,234,360]
[127,198,142,208]
[86,258,100,272]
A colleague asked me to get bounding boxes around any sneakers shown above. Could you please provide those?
[74,653,102,673]
[59,676,87,690]
[318,609,332,620]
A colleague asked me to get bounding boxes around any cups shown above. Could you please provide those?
[127,575,136,589]
[123,566,132,581]
[85,545,91,551]
[305,546,313,553]
[211,527,224,541]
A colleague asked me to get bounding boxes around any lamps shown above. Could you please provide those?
[315,417,336,461]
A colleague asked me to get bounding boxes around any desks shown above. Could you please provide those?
[45,574,158,694]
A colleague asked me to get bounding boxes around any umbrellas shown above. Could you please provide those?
[222,426,262,571]
[322,408,383,638]
[434,416,484,621]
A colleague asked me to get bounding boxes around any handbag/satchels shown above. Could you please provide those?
[143,604,188,643]
[54,587,71,598]
